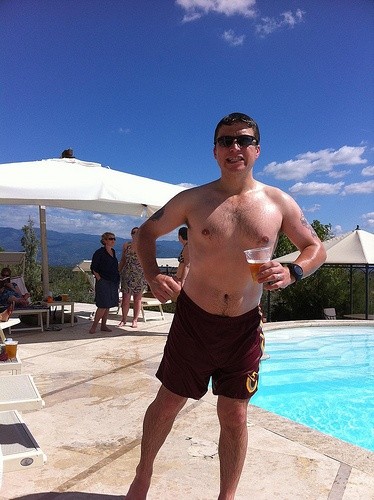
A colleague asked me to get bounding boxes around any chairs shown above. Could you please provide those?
[0,275,49,474]
[90,274,172,322]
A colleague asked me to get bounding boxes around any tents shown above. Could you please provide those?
[270,230,374,319]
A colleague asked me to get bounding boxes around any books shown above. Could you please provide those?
[0,277,10,288]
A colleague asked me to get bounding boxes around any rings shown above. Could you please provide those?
[277,274,280,280]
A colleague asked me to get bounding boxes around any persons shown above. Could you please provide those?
[126,112,327,500]
[176,226,191,289]
[115,226,147,329]
[89,231,121,334]
[0,268,31,307]
[0,303,14,322]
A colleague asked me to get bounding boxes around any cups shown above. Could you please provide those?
[5,341,18,360]
[243,247,270,283]
[62,294,67,302]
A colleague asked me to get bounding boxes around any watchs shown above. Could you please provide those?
[286,263,304,282]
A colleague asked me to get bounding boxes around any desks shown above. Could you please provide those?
[37,301,74,327]
[0,355,21,375]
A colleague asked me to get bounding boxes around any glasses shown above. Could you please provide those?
[217,134,257,147]
[109,237,116,240]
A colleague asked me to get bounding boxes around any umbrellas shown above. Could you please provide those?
[0,158,190,219]
